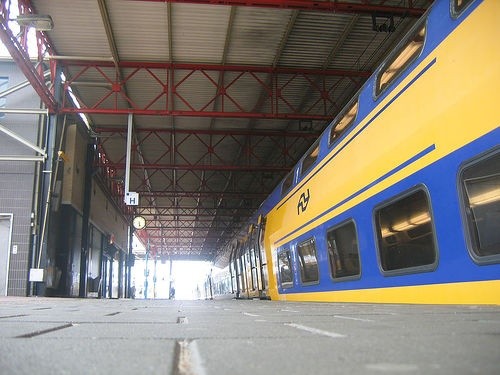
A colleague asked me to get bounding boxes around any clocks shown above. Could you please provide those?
[133,216,147,230]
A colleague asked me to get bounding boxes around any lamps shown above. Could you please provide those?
[8,14,54,31]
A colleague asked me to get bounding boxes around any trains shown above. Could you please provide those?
[196,0,500,305]
